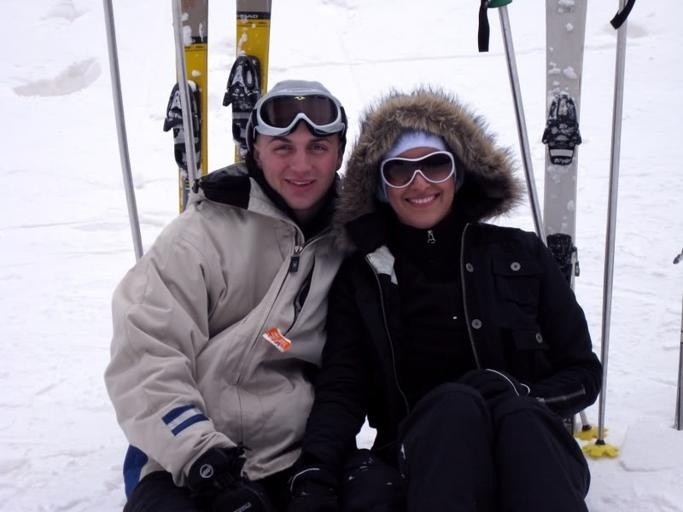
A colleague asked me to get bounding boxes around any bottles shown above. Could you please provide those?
[382,131,457,201]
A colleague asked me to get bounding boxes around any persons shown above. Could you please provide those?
[103,81,348,512]
[271,92,603,512]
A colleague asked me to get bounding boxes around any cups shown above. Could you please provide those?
[380,150,458,189]
[246,90,347,142]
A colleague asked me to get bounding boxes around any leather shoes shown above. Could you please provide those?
[186,441,262,511]
[287,447,343,512]
[461,364,532,413]
